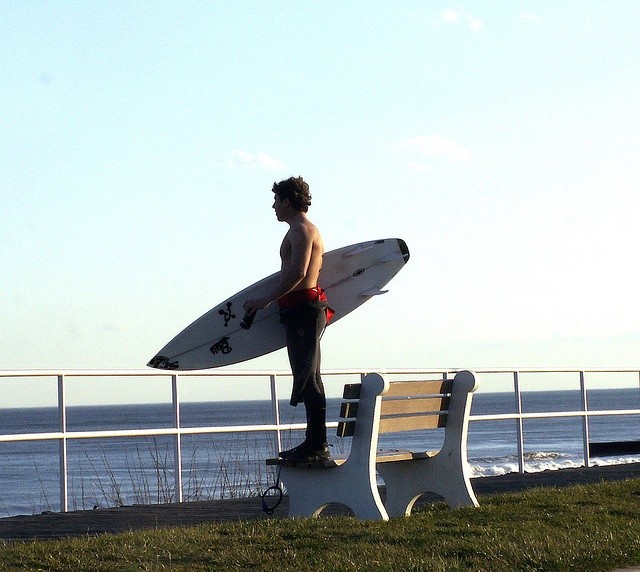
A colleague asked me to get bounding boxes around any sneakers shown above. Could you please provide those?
[278,440,330,459]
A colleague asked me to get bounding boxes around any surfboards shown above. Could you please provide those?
[147,237,410,370]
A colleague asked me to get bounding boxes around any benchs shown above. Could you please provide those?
[266,369,481,522]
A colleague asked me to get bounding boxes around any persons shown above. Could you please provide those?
[242,176,334,463]
